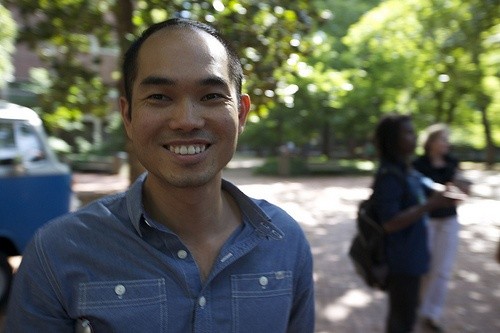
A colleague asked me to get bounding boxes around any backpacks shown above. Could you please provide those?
[349,200,390,289]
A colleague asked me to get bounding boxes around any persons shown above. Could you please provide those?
[373,116,463,332]
[0,15,316,332]
[408,125,497,332]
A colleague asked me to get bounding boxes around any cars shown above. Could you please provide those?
[0,102,74,312]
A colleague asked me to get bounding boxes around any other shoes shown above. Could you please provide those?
[421,319,442,333]
